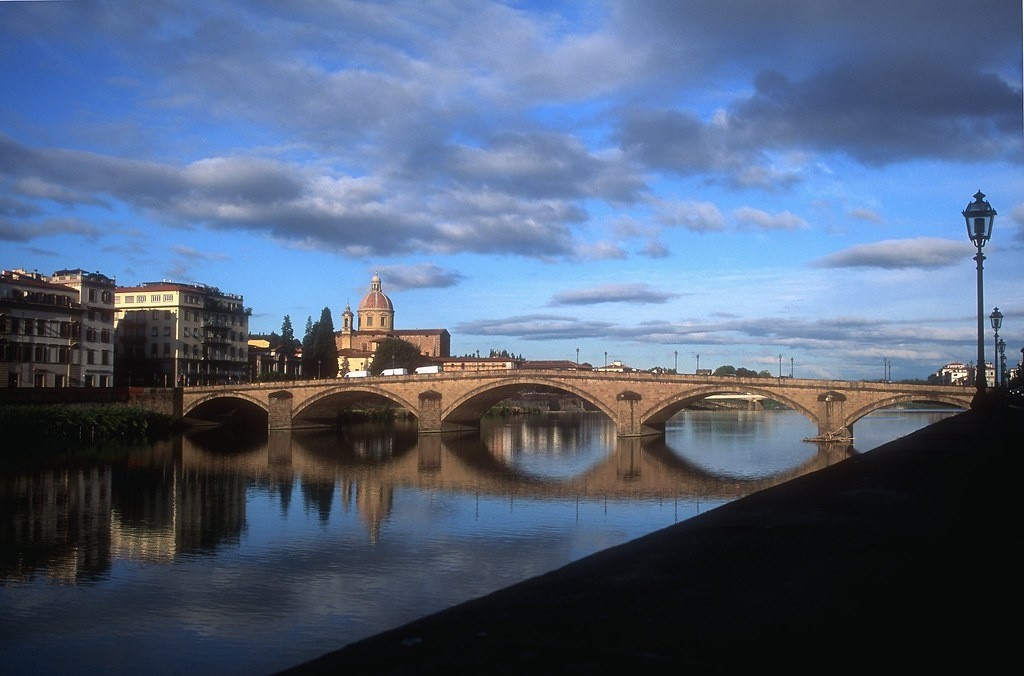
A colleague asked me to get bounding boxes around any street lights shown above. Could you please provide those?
[391,354,395,376]
[962,188,998,410]
[604,351,607,372]
[998,338,1007,389]
[888,360,891,381]
[696,354,699,370]
[576,348,579,371]
[883,357,887,382]
[778,354,782,378]
[791,357,794,378]
[318,360,321,380]
[988,307,1004,390]
[674,350,678,374]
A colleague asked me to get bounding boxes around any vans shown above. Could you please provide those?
[379,368,408,375]
[413,366,438,374]
[344,371,371,377]
